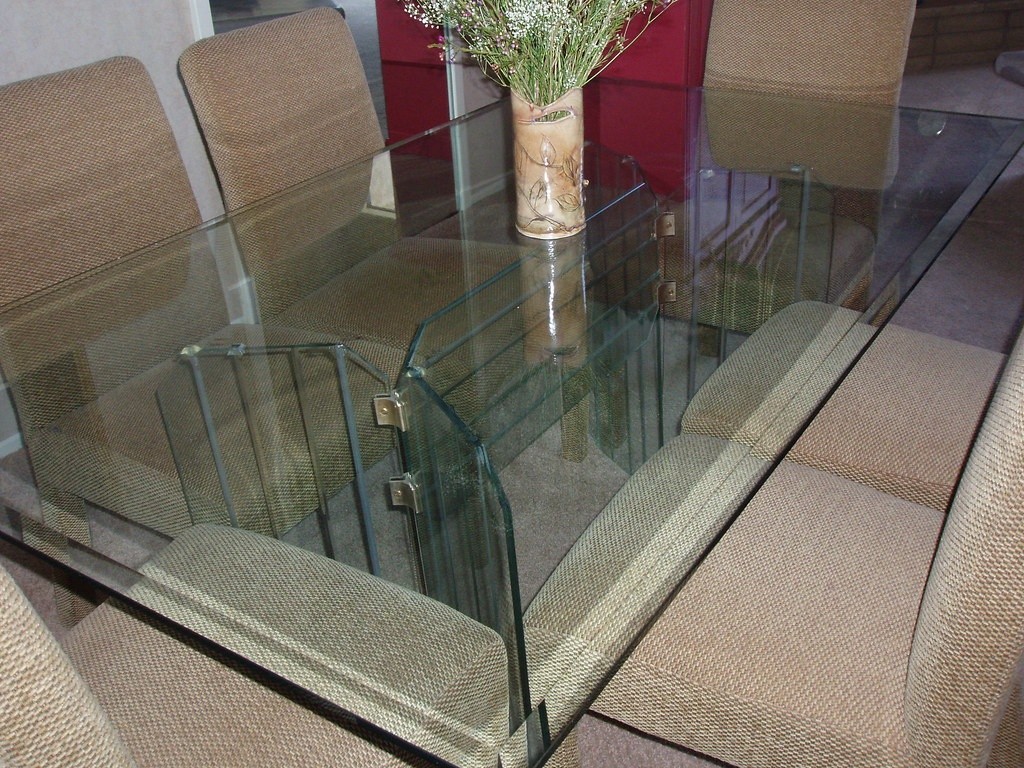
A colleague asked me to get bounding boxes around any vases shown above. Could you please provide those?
[509,86,586,239]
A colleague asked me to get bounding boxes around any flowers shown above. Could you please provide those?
[397,0,678,122]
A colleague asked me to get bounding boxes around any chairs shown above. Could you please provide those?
[521,330,1024,768]
[680,297,1024,513]
[600,0,915,442]
[0,55,483,622]
[0,524,507,768]
[177,7,653,621]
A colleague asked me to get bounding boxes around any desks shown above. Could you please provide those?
[0,79,1024,768]
